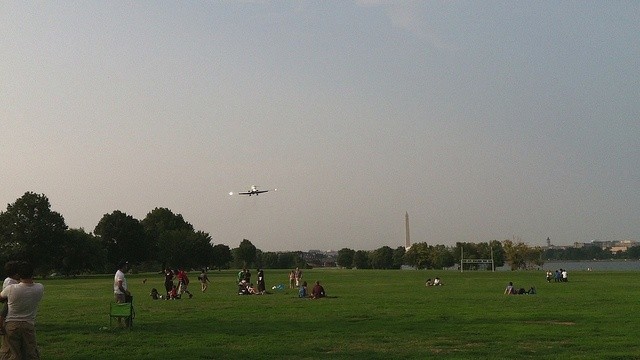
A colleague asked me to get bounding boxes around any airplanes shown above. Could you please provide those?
[230,185,278,196]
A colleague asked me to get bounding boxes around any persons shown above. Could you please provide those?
[298,281,308,298]
[310,280,326,299]
[164,266,176,297]
[1,261,21,336]
[294,266,303,287]
[238,267,245,282]
[176,264,193,300]
[256,267,266,295]
[199,270,209,292]
[239,280,248,295]
[168,285,178,300]
[1,262,44,360]
[546,271,553,283]
[503,281,524,295]
[149,288,162,299]
[434,277,440,285]
[424,278,434,286]
[113,258,131,329]
[246,284,256,295]
[288,270,295,289]
[554,268,568,284]
[245,269,251,286]
